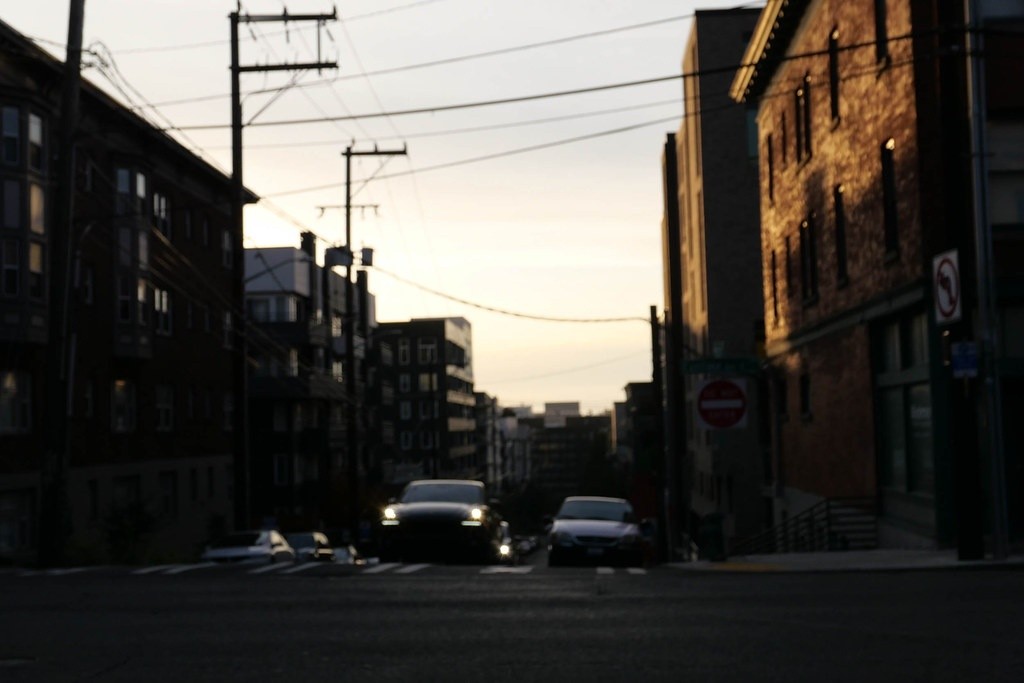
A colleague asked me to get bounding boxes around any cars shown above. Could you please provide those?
[333,544,379,566]
[377,478,538,569]
[201,528,294,564]
[544,498,649,562]
[284,532,335,563]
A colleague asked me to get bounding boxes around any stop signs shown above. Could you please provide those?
[693,381,748,432]
